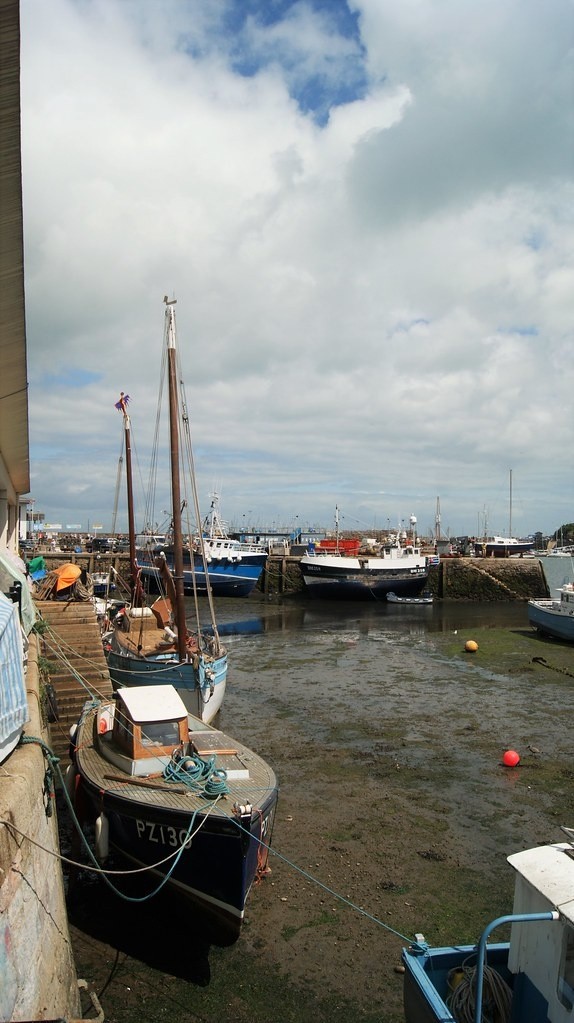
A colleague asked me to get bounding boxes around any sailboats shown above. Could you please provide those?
[101,292,230,727]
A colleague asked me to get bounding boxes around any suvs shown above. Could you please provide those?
[85,537,118,553]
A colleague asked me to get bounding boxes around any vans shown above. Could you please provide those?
[135,535,166,551]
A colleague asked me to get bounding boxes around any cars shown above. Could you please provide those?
[19,539,37,552]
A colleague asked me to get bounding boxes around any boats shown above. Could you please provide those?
[401,826,574,1023]
[298,499,433,600]
[135,473,269,597]
[527,581,574,645]
[69,683,279,938]
[474,468,534,557]
[92,572,117,597]
